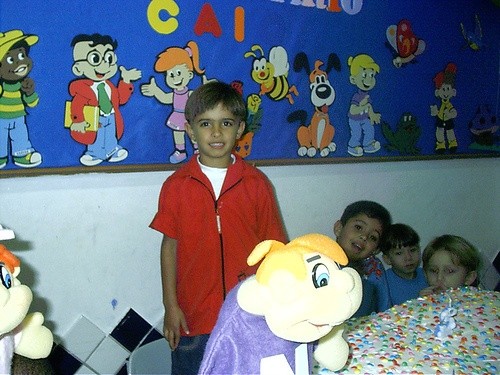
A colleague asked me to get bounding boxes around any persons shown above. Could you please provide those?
[148,81,290,375]
[333,200,480,318]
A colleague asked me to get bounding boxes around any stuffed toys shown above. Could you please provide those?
[197,232,363,375]
[0,224,54,375]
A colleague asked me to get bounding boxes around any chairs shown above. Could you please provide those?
[129,338,172,375]
